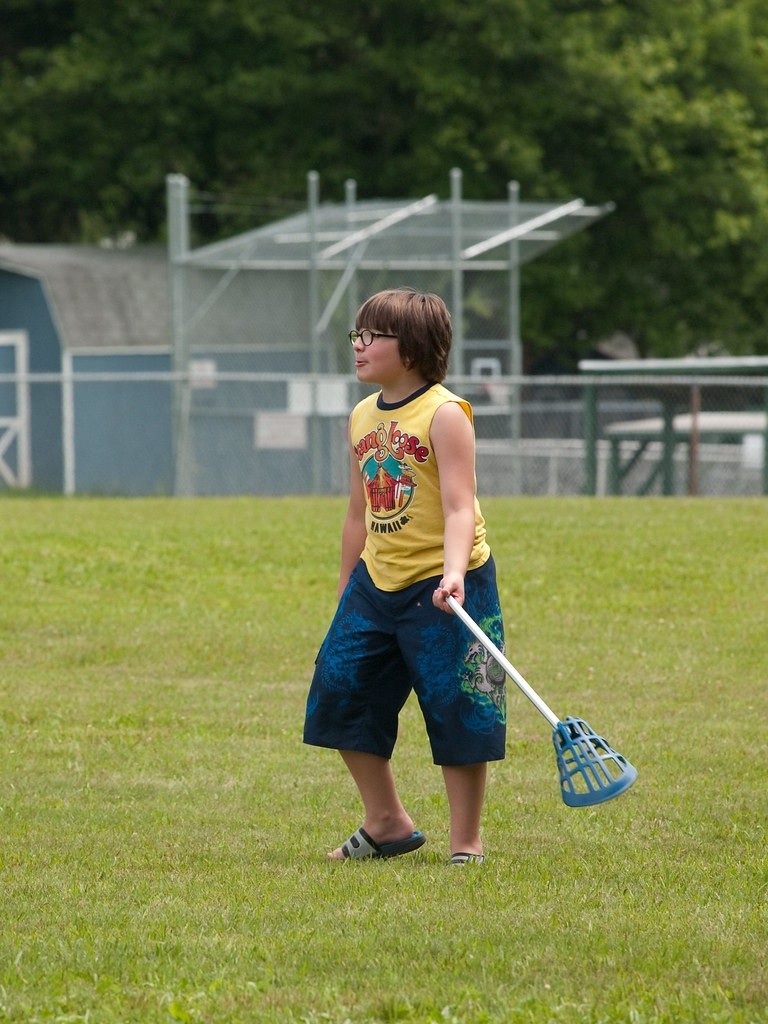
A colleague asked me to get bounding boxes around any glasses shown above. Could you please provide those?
[349,329,399,346]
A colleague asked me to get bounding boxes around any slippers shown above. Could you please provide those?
[451,852,485,867]
[341,825,427,861]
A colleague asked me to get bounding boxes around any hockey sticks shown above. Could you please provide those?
[436,586,639,808]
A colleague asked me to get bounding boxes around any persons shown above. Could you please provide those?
[303,289,507,865]
